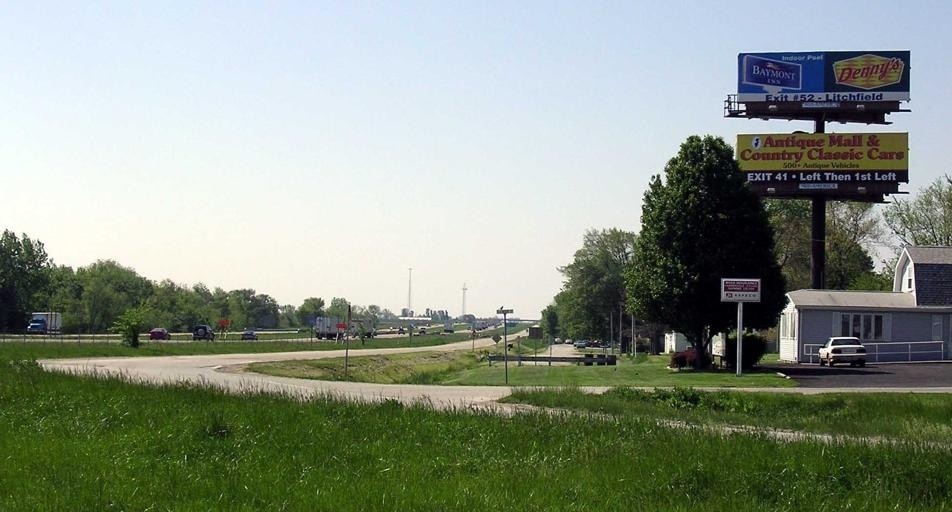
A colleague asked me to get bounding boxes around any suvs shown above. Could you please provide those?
[192,325,215,341]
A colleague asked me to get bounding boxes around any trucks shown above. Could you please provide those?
[315,316,348,340]
[351,318,377,339]
[27,312,62,335]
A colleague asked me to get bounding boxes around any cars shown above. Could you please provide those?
[149,328,171,340]
[398,319,502,335]
[240,331,257,340]
[818,336,867,367]
[554,338,611,349]
